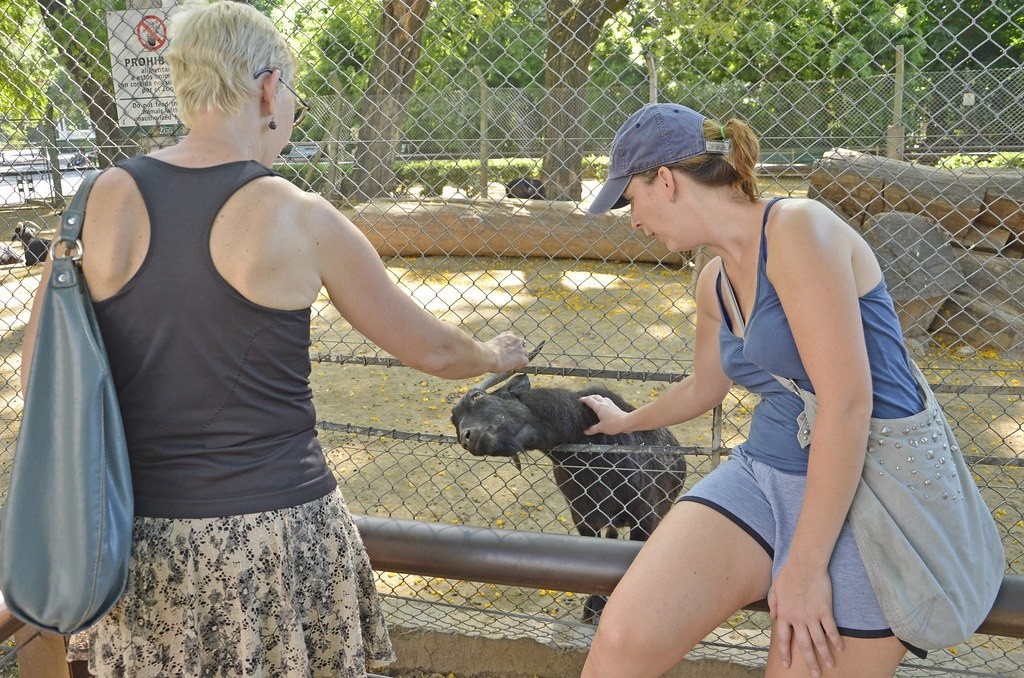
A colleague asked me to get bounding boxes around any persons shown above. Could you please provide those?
[19,1,530,678]
[578,102,959,677]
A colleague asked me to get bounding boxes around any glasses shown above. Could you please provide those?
[254,68,311,128]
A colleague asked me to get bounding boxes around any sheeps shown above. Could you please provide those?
[452,341,686,624]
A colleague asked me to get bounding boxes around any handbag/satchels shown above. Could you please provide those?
[0,169,134,636]
[796,388,1007,649]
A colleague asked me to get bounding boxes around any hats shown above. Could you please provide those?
[588,103,730,215]
